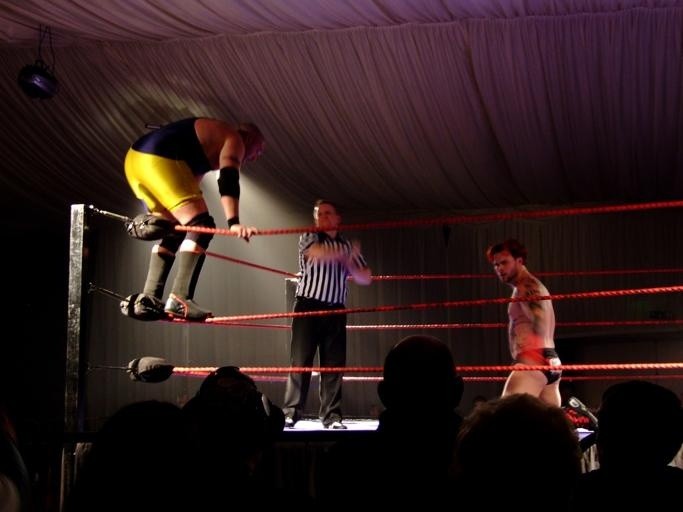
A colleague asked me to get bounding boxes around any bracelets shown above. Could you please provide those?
[227,216,239,229]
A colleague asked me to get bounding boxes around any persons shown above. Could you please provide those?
[282,200,371,430]
[0,367,285,512]
[376,336,462,507]
[471,397,485,412]
[571,380,683,510]
[283,431,379,511]
[124,116,267,323]
[451,394,581,508]
[486,240,600,428]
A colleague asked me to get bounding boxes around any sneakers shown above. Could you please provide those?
[324,422,347,429]
[164,293,212,320]
[284,417,295,427]
